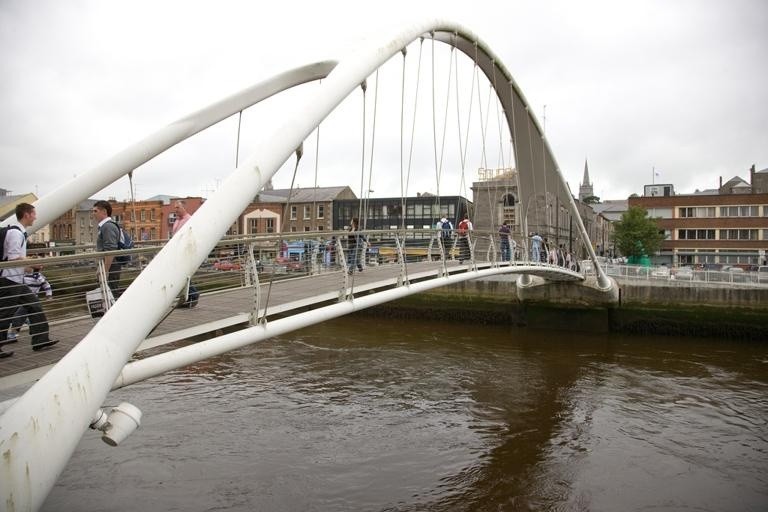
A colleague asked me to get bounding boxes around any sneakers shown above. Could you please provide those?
[8,334,18,344]
[176,291,200,309]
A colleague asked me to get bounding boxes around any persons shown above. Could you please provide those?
[92,199,124,312]
[0,202,59,360]
[346,217,371,275]
[457,214,473,264]
[498,222,621,274]
[436,212,454,264]
[1,251,55,345]
[315,234,337,268]
[169,198,200,307]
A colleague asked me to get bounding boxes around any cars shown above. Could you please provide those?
[581,260,767,282]
[200,258,264,270]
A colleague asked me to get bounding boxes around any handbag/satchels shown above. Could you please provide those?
[86,286,117,319]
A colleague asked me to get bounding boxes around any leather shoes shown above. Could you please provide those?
[32,339,59,351]
[0,350,14,358]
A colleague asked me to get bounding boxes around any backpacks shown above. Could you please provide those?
[104,220,134,266]
[0,225,25,277]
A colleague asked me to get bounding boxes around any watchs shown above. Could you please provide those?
[366,240,369,243]
